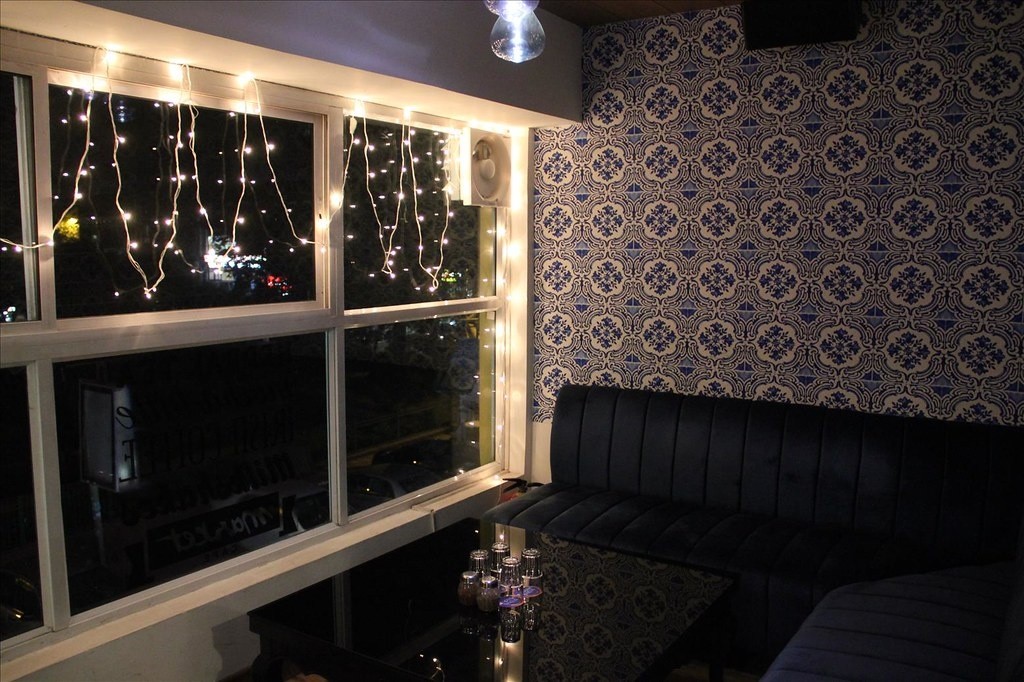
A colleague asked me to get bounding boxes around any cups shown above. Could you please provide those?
[460,539,545,628]
[460,597,540,643]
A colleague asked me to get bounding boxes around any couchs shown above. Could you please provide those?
[759,558,1024,682]
[479,380,1024,673]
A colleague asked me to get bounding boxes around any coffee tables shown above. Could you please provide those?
[248,517,746,682]
